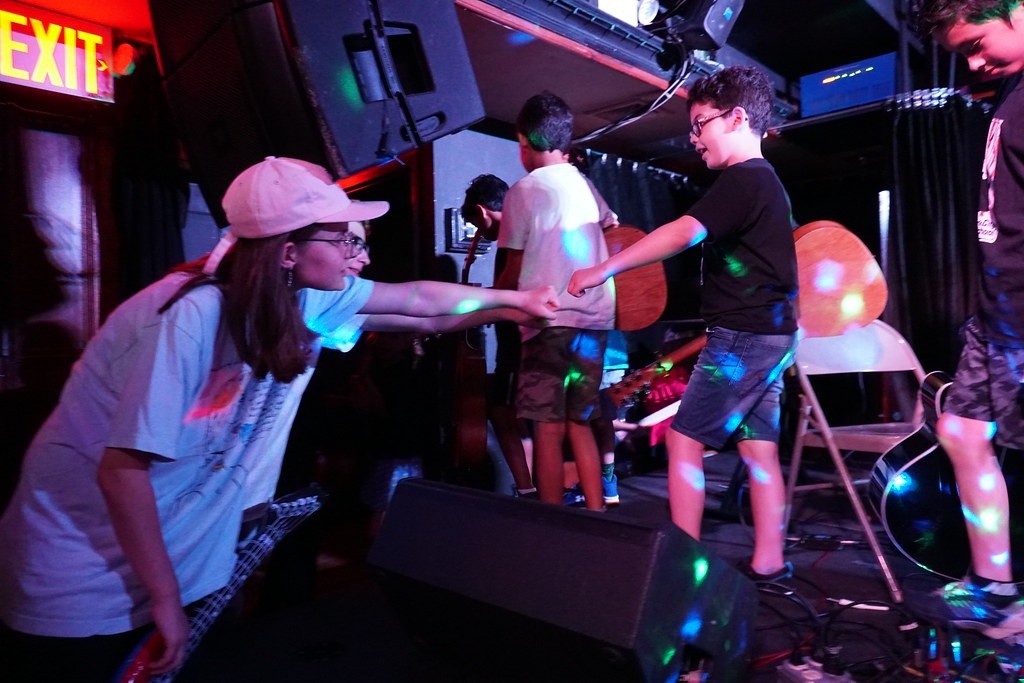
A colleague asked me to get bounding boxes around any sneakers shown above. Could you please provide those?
[561,485,586,510]
[601,477,619,503]
[907,575,1024,640]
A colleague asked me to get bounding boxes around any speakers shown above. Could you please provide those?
[148,0,485,237]
[368,476,755,683]
[681,0,745,51]
[415,128,531,375]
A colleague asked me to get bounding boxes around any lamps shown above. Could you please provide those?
[677,1,745,51]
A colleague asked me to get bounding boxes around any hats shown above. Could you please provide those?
[222,156,390,239]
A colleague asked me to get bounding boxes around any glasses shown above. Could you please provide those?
[689,108,747,137]
[349,236,369,257]
[294,236,354,259]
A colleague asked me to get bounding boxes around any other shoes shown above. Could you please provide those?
[743,564,791,595]
[518,490,537,499]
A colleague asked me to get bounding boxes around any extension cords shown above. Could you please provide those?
[775,655,858,683]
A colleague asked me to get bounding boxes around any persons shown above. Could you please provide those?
[903,0,1024,643]
[0,90,618,681]
[566,64,803,598]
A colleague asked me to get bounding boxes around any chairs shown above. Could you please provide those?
[783,319,925,603]
